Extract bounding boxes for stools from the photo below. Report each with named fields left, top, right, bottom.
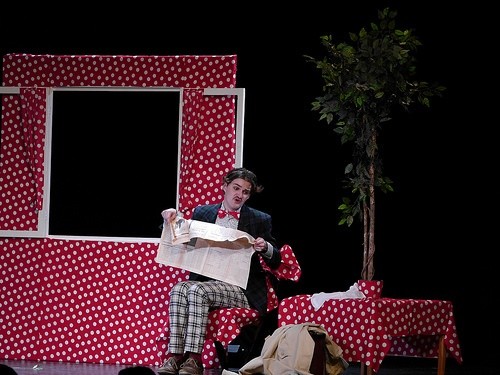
left=308, top=331, right=327, bottom=375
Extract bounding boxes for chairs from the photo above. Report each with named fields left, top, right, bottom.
left=153, top=246, right=300, bottom=375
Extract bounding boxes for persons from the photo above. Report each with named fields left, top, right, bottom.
left=158, top=168, right=282, bottom=375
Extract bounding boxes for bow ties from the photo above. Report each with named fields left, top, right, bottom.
left=217, top=208, right=240, bottom=220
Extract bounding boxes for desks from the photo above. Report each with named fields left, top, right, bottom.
left=278, top=293, right=463, bottom=375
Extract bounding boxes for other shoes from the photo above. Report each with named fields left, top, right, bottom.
left=178, top=357, right=204, bottom=375
left=157, top=355, right=183, bottom=374
